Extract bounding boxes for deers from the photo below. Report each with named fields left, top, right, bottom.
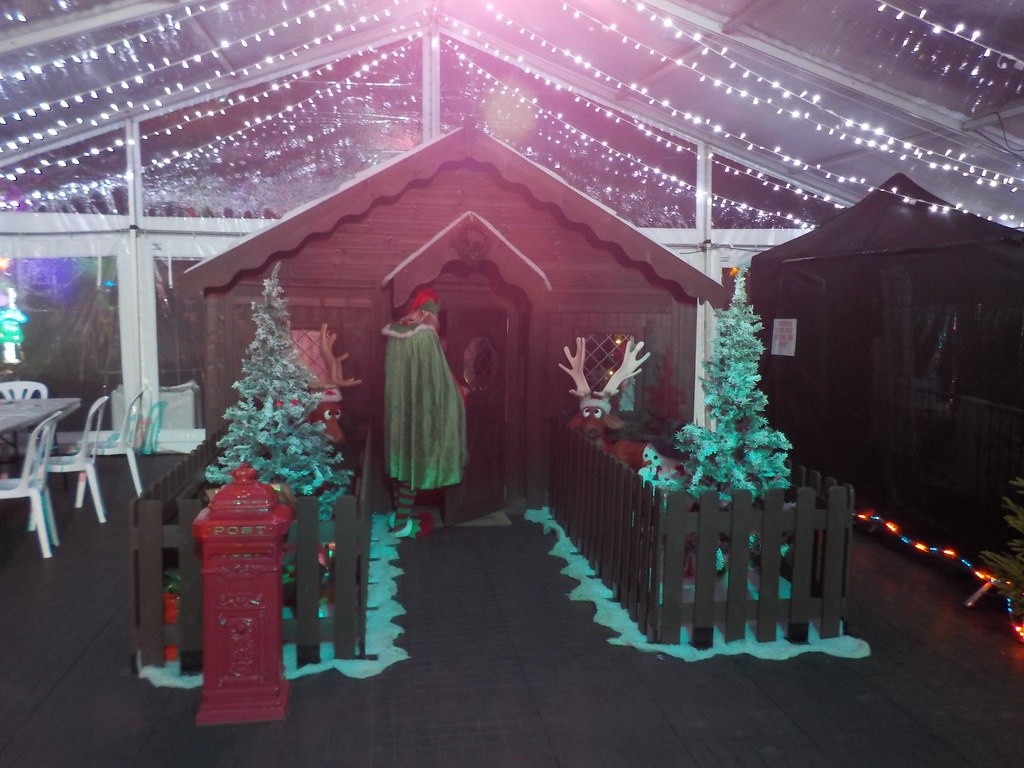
left=277, top=323, right=363, bottom=445
left=557, top=336, right=686, bottom=479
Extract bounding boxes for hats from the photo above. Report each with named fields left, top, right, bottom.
left=412, top=287, right=440, bottom=313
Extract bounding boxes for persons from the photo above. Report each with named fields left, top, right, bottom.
left=381, top=288, right=469, bottom=538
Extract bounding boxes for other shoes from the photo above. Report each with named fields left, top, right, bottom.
left=392, top=513, right=433, bottom=542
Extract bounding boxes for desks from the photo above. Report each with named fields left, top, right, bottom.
left=0, top=398, right=82, bottom=492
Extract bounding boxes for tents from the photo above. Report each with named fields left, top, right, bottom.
left=751, top=173, right=1024, bottom=590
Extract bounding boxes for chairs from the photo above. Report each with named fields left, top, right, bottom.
left=27, top=395, right=110, bottom=532
left=0, top=409, right=64, bottom=559
left=0, top=380, right=49, bottom=454
left=74, top=387, right=147, bottom=509
